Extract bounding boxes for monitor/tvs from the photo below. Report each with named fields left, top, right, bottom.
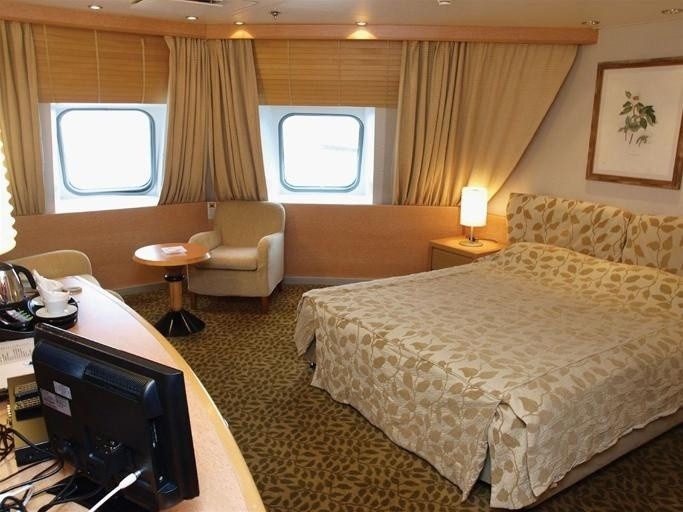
left=31, top=322, right=201, bottom=512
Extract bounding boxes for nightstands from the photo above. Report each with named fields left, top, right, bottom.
left=427, top=234, right=504, bottom=275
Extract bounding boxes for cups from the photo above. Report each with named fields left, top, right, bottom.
left=42, top=291, right=71, bottom=313
left=36, top=286, right=61, bottom=302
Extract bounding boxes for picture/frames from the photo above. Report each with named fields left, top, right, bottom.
left=588, top=56, right=683, bottom=192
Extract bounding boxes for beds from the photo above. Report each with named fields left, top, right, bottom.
left=293, top=193, right=683, bottom=507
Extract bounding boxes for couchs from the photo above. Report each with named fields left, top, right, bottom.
left=184, top=195, right=286, bottom=316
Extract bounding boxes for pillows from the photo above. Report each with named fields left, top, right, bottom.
left=623, top=214, right=683, bottom=277
left=507, top=188, right=636, bottom=267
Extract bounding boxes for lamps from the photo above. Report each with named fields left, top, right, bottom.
left=461, top=186, right=485, bottom=247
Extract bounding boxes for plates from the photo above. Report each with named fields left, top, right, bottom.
left=36, top=305, right=77, bottom=318
left=26, top=295, right=79, bottom=330
left=31, top=296, right=46, bottom=307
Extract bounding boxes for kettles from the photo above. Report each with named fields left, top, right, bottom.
left=0, top=260, right=38, bottom=313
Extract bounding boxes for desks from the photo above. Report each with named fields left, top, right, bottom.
left=0, top=245, right=268, bottom=508
left=131, top=241, right=212, bottom=337
